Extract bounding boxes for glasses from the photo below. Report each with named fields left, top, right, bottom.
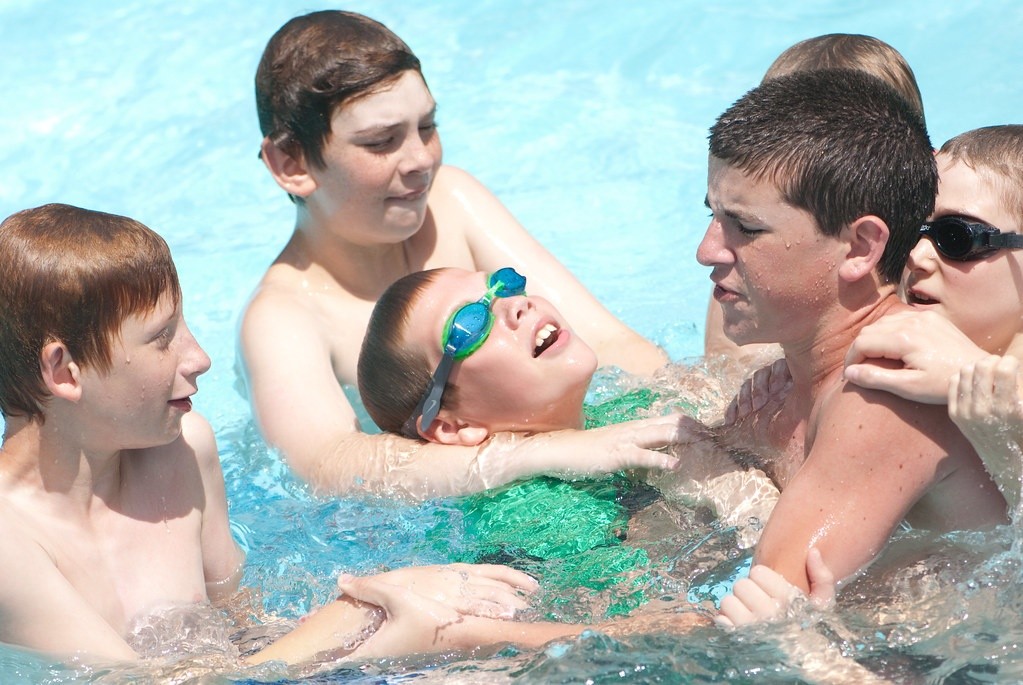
left=918, top=215, right=1023, bottom=262
left=442, top=267, right=527, bottom=361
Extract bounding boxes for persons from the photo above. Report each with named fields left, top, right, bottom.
left=0, top=203, right=540, bottom=669
left=706, top=34, right=1022, bottom=630
left=335, top=69, right=1012, bottom=659
left=358, top=267, right=595, bottom=444
left=241, top=9, right=708, bottom=495
